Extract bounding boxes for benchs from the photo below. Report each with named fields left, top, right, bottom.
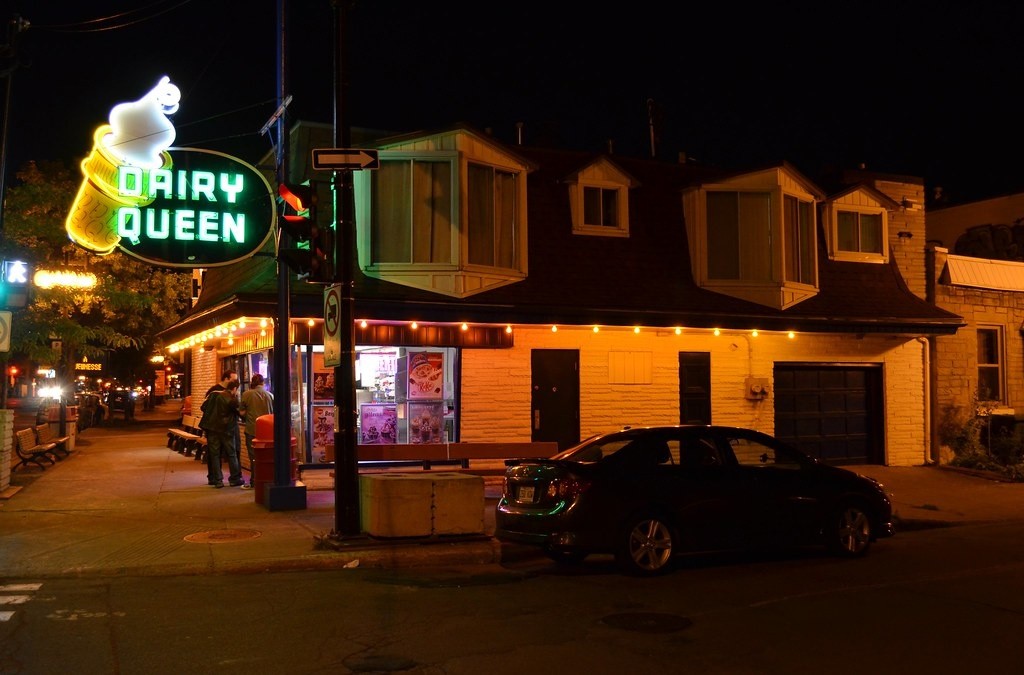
left=33, top=423, right=71, bottom=461
left=325, top=442, right=558, bottom=504
left=11, top=428, right=56, bottom=473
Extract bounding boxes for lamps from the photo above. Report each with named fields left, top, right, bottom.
left=192, top=278, right=201, bottom=299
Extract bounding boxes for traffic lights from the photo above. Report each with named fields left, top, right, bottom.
left=10, top=358, right=17, bottom=375
left=166, top=367, right=172, bottom=377
left=275, top=181, right=338, bottom=284
left=0, top=255, right=33, bottom=312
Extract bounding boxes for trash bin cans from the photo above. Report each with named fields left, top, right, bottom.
left=250, top=414, right=299, bottom=506
left=48, top=405, right=76, bottom=451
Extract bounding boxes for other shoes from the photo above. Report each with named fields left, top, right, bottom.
left=240, top=484, right=255, bottom=489
left=230, top=478, right=245, bottom=486
left=215, top=480, right=224, bottom=487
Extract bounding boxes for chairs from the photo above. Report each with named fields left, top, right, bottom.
left=167, top=415, right=207, bottom=464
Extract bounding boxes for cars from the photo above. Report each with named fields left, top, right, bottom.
left=493, top=425, right=894, bottom=577
left=36, top=390, right=105, bottom=433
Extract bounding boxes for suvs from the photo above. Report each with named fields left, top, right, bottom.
left=108, top=391, right=133, bottom=410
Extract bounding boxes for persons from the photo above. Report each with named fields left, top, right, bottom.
left=203, top=370, right=243, bottom=485
left=239, top=373, right=274, bottom=489
left=198, top=379, right=249, bottom=489
